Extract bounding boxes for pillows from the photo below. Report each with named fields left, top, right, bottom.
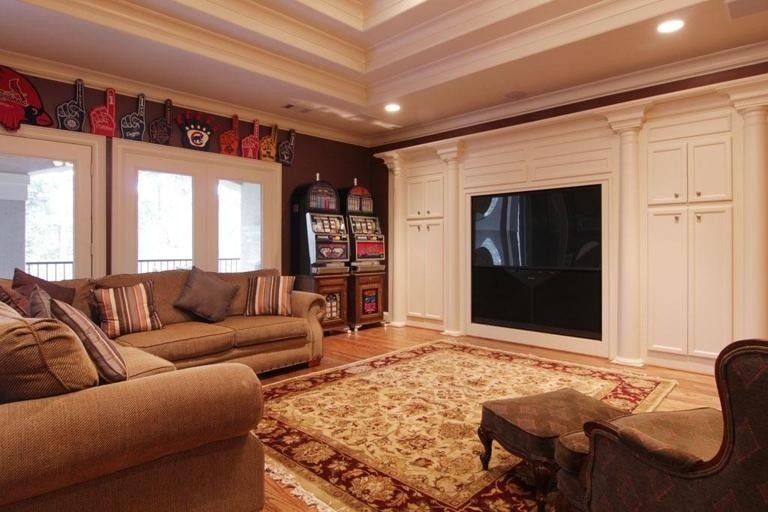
left=0, top=282, right=30, bottom=317
left=173, top=266, right=240, bottom=323
left=27, top=283, right=55, bottom=320
left=243, top=274, right=295, bottom=316
left=91, top=279, right=162, bottom=340
left=47, top=298, right=129, bottom=382
left=12, top=267, right=76, bottom=305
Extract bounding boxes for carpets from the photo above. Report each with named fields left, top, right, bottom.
left=247, top=340, right=678, bottom=511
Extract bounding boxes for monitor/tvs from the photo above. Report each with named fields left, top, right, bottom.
left=472, top=185, right=601, bottom=271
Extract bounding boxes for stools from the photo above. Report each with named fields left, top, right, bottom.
left=477, top=389, right=637, bottom=511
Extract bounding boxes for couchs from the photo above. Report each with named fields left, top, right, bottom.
left=90, top=269, right=327, bottom=375
left=0, top=278, right=264, bottom=512
left=545, top=339, right=768, bottom=512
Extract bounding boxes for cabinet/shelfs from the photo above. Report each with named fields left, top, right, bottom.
left=349, top=272, right=387, bottom=333
left=644, top=132, right=736, bottom=369
left=293, top=275, right=352, bottom=336
left=405, top=173, right=447, bottom=333
